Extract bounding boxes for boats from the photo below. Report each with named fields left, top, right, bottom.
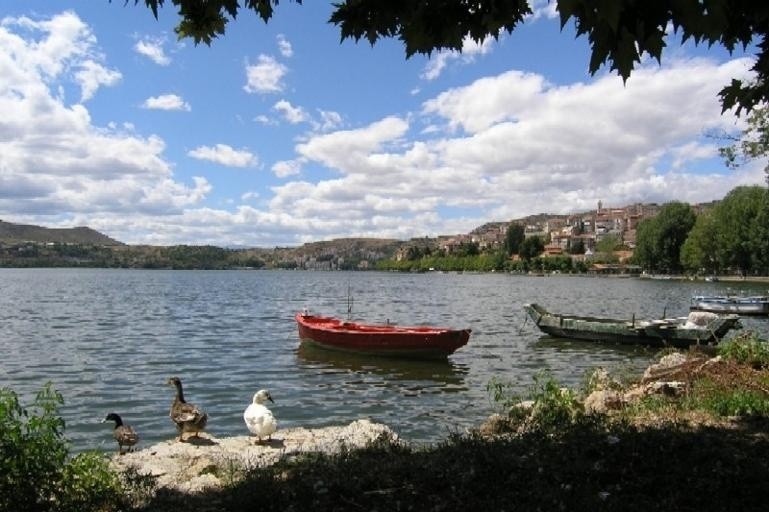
left=293, top=308, right=471, bottom=359
left=520, top=302, right=739, bottom=349
left=690, top=287, right=769, bottom=320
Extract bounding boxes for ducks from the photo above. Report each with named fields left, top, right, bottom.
left=242, top=389, right=279, bottom=445
left=167, top=377, right=207, bottom=443
left=101, top=412, right=140, bottom=454
